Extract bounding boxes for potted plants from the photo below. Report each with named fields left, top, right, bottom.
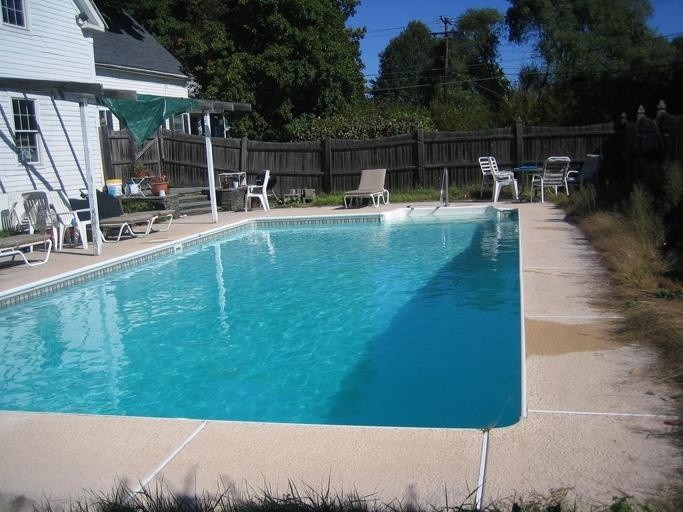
left=134, top=164, right=145, bottom=177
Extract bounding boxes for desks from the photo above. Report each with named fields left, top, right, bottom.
left=512, top=166, right=542, bottom=198
left=219, top=172, right=246, bottom=188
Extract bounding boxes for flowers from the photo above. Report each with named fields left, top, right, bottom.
left=151, top=175, right=168, bottom=183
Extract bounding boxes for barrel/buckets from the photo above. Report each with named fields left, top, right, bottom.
left=106, top=179, right=123, bottom=196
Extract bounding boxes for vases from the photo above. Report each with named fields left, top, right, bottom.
left=151, top=182, right=168, bottom=196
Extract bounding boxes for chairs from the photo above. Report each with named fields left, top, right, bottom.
left=344, top=169, right=389, bottom=207
left=530, top=156, right=570, bottom=204
left=245, top=170, right=270, bottom=212
left=567, top=154, right=601, bottom=191
left=0, top=188, right=175, bottom=266
left=479, top=157, right=518, bottom=203
